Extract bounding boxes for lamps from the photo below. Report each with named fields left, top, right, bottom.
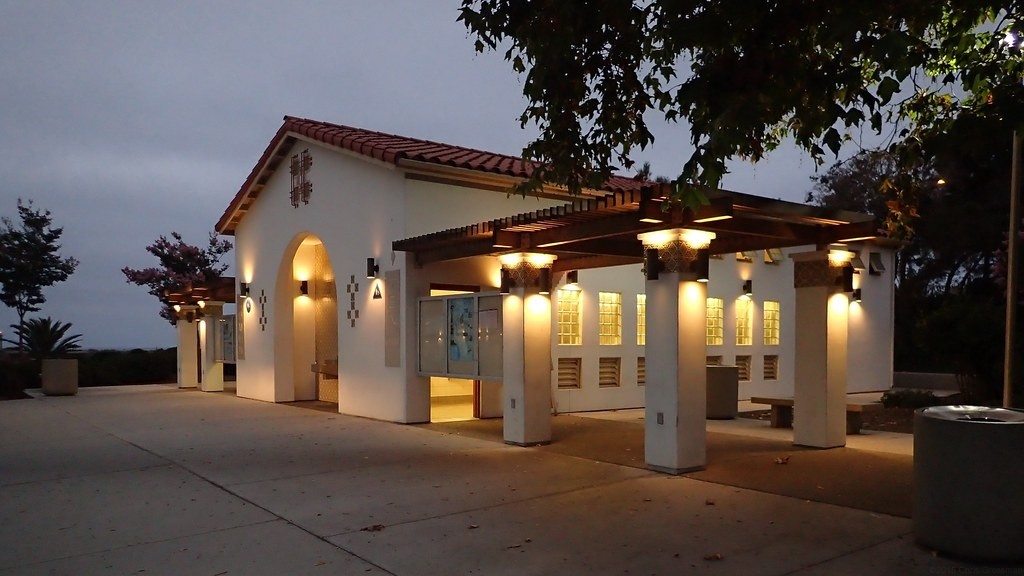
left=366, top=257, right=379, bottom=279
left=568, top=269, right=579, bottom=285
left=538, top=268, right=550, bottom=295
left=647, top=248, right=665, bottom=281
left=195, top=308, right=205, bottom=322
left=841, top=264, right=862, bottom=306
left=299, top=281, right=308, bottom=294
left=499, top=268, right=516, bottom=295
left=240, top=283, right=250, bottom=297
left=742, top=280, right=753, bottom=296
left=691, top=248, right=709, bottom=282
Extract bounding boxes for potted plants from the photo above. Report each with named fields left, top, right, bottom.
left=0, top=315, right=82, bottom=395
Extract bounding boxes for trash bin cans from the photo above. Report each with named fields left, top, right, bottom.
left=706, top=364, right=738, bottom=420
left=911, top=403, right=1024, bottom=563
left=41, top=358, right=78, bottom=394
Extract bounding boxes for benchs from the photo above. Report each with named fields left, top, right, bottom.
left=751, top=397, right=884, bottom=437
